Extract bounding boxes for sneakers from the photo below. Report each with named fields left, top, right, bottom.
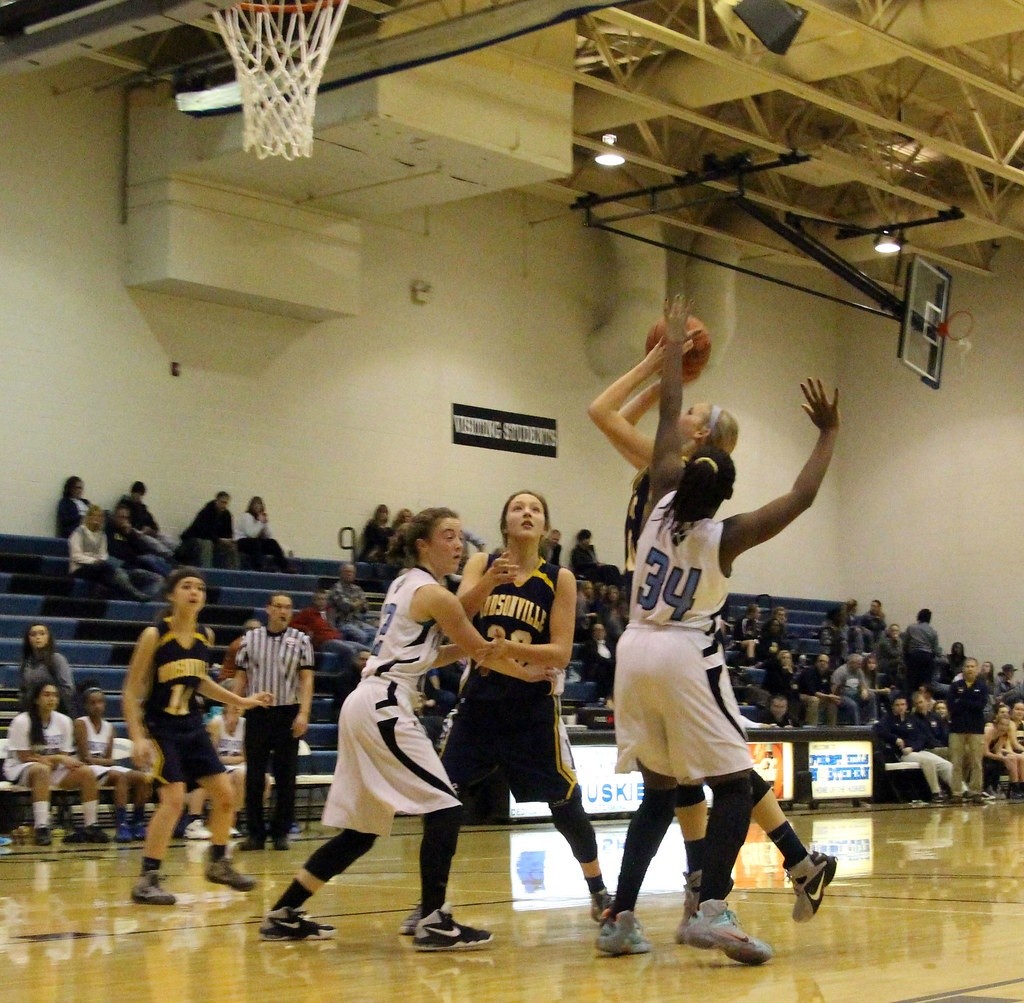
left=595, top=910, right=652, bottom=953
left=274, top=838, right=289, bottom=850
left=132, top=869, right=177, bottom=905
left=784, top=851, right=838, bottom=922
left=206, top=856, right=254, bottom=890
left=677, top=870, right=735, bottom=940
left=684, top=899, right=773, bottom=964
left=35, top=827, right=51, bottom=846
left=239, top=838, right=264, bottom=851
left=411, top=903, right=493, bottom=949
left=260, top=905, right=339, bottom=941
left=589, top=898, right=642, bottom=929
left=117, top=822, right=132, bottom=842
left=82, top=824, right=109, bottom=843
left=133, top=823, right=146, bottom=839
left=399, top=903, right=452, bottom=933
left=184, top=819, right=212, bottom=839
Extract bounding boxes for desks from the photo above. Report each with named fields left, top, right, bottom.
left=483, top=729, right=878, bottom=823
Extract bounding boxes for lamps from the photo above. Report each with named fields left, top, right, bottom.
left=731, top=0, right=811, bottom=59
left=171, top=60, right=243, bottom=117
left=874, top=229, right=900, bottom=255
left=593, top=131, right=626, bottom=167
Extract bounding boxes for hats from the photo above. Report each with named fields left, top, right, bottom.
left=1003, top=664, right=1017, bottom=672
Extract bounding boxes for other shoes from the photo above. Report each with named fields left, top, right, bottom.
left=290, top=824, right=301, bottom=834
left=0, top=837, right=12, bottom=846
left=931, top=785, right=1023, bottom=804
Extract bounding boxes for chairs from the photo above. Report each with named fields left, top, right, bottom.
left=294, top=740, right=335, bottom=832
left=869, top=720, right=922, bottom=803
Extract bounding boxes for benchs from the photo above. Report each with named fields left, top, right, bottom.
left=0, top=532, right=863, bottom=770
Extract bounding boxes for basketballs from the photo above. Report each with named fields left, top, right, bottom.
left=644, top=313, right=711, bottom=383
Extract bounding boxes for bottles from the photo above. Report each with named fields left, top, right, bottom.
left=289, top=550, right=293, bottom=558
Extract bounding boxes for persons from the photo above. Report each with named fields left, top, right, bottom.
left=259, top=509, right=559, bottom=949
left=226, top=595, right=313, bottom=851
left=415, top=529, right=487, bottom=747
left=4, top=617, right=309, bottom=844
left=721, top=595, right=1024, bottom=805
left=589, top=327, right=836, bottom=941
left=330, top=652, right=370, bottom=721
left=290, top=563, right=377, bottom=666
left=233, top=495, right=299, bottom=573
left=122, top=568, right=274, bottom=904
left=57, top=475, right=219, bottom=602
left=184, top=493, right=241, bottom=570
left=595, top=292, right=839, bottom=963
left=360, top=504, right=415, bottom=590
left=544, top=527, right=631, bottom=704
left=402, top=491, right=642, bottom=934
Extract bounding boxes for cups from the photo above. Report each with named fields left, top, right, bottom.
left=566, top=716, right=577, bottom=725
